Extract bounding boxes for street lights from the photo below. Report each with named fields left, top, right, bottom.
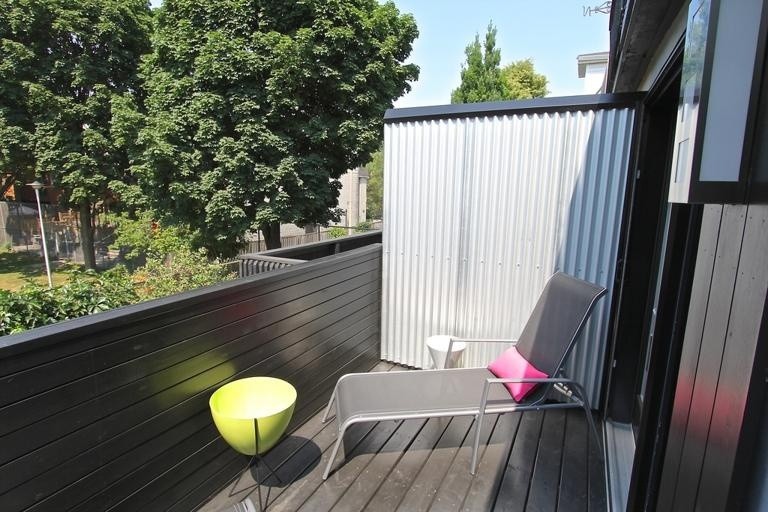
left=23, top=179, right=55, bottom=292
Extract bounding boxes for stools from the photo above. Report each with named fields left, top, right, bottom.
left=208, top=375, right=297, bottom=512
left=426, top=334, right=465, bottom=368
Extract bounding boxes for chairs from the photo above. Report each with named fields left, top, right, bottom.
left=322, top=273, right=606, bottom=479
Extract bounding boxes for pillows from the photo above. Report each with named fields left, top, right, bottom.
left=487, top=343, right=548, bottom=403
